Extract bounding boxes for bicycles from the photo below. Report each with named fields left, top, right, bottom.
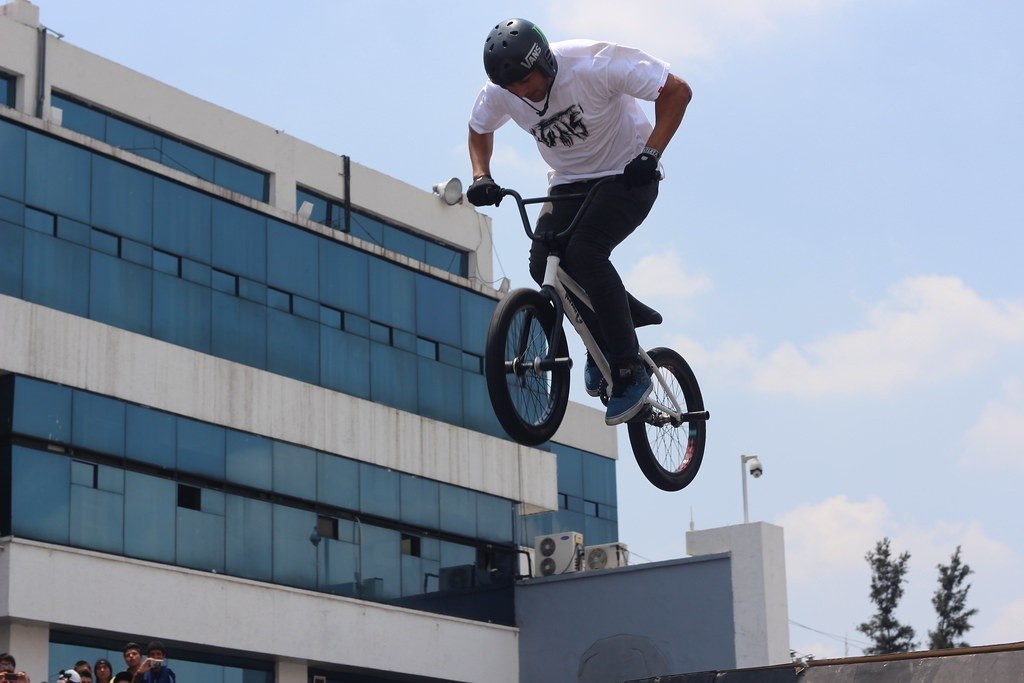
left=485, top=168, right=715, bottom=495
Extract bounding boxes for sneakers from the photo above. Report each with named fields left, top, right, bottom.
left=584, top=346, right=606, bottom=397
left=605, top=365, right=654, bottom=425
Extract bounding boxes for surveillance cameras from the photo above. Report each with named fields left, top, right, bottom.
left=749, top=460, right=764, bottom=478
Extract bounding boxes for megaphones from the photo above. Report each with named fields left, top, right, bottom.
left=433, top=177, right=463, bottom=205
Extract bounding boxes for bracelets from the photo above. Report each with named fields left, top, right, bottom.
left=642, top=147, right=661, bottom=161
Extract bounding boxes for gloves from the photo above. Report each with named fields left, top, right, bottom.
left=467, top=174, right=500, bottom=207
left=624, top=147, right=662, bottom=184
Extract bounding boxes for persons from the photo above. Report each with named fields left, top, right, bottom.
left=59, top=642, right=175, bottom=683
left=0, top=653, right=29, bottom=683
left=468, top=18, right=693, bottom=426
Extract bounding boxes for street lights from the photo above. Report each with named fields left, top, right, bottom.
left=741, top=453, right=764, bottom=523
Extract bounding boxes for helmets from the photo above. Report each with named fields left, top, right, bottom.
left=484, top=18, right=557, bottom=85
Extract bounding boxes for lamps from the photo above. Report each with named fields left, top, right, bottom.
left=50, top=104, right=64, bottom=127
left=498, top=277, right=511, bottom=294
left=298, top=200, right=315, bottom=219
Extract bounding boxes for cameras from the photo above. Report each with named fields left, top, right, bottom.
left=150, top=659, right=165, bottom=666
left=4, top=673, right=19, bottom=680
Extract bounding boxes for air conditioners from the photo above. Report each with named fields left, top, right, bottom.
left=439, top=565, right=475, bottom=589
left=535, top=530, right=585, bottom=578
left=582, top=542, right=628, bottom=570
